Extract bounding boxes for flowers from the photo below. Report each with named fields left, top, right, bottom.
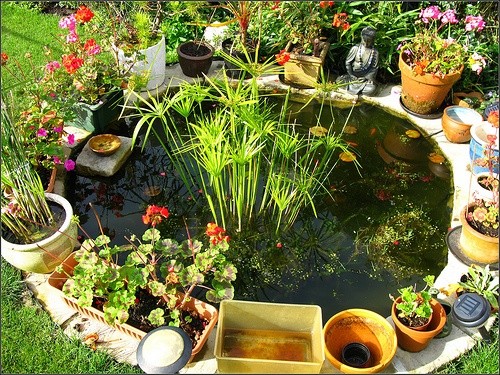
left=0, top=1, right=150, bottom=236
left=186, top=1, right=349, bottom=55
left=464, top=111, right=499, bottom=230
left=1, top=201, right=238, bottom=327
left=398, top=3, right=489, bottom=78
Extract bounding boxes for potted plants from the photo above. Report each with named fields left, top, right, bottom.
left=440, top=262, right=499, bottom=312
left=169, top=1, right=216, bottom=78
left=389, top=273, right=440, bottom=330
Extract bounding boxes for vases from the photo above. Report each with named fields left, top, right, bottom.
left=47, top=251, right=218, bottom=363
left=47, top=79, right=125, bottom=134
left=442, top=104, right=485, bottom=144
left=471, top=170, right=500, bottom=203
left=322, top=307, right=398, bottom=375
left=3, top=113, right=65, bottom=198
left=391, top=294, right=447, bottom=354
left=222, top=36, right=260, bottom=78
left=468, top=120, right=499, bottom=175
left=110, top=28, right=166, bottom=92
left=1, top=194, right=77, bottom=274
left=460, top=199, right=499, bottom=264
left=283, top=38, right=330, bottom=87
left=398, top=49, right=466, bottom=113
